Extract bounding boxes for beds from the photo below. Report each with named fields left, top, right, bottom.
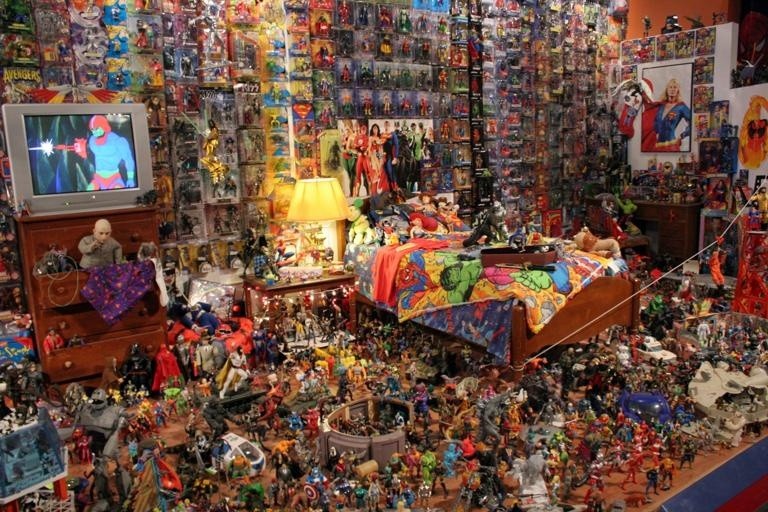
left=338, top=191, right=641, bottom=384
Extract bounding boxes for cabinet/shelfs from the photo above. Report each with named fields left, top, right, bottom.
left=13, top=203, right=169, bottom=392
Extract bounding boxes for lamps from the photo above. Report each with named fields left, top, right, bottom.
left=286, top=177, right=352, bottom=272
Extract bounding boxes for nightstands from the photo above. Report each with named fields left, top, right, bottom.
left=242, top=273, right=356, bottom=336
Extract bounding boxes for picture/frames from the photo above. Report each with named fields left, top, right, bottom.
left=641, top=62, right=694, bottom=153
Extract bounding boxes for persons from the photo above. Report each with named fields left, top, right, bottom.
left=1, top=0, right=768, bottom=512
left=0, top=1, right=767, bottom=511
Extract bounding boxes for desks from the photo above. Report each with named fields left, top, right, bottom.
left=621, top=198, right=703, bottom=263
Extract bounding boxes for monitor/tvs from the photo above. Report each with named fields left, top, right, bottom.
left=1, top=103, right=154, bottom=217
